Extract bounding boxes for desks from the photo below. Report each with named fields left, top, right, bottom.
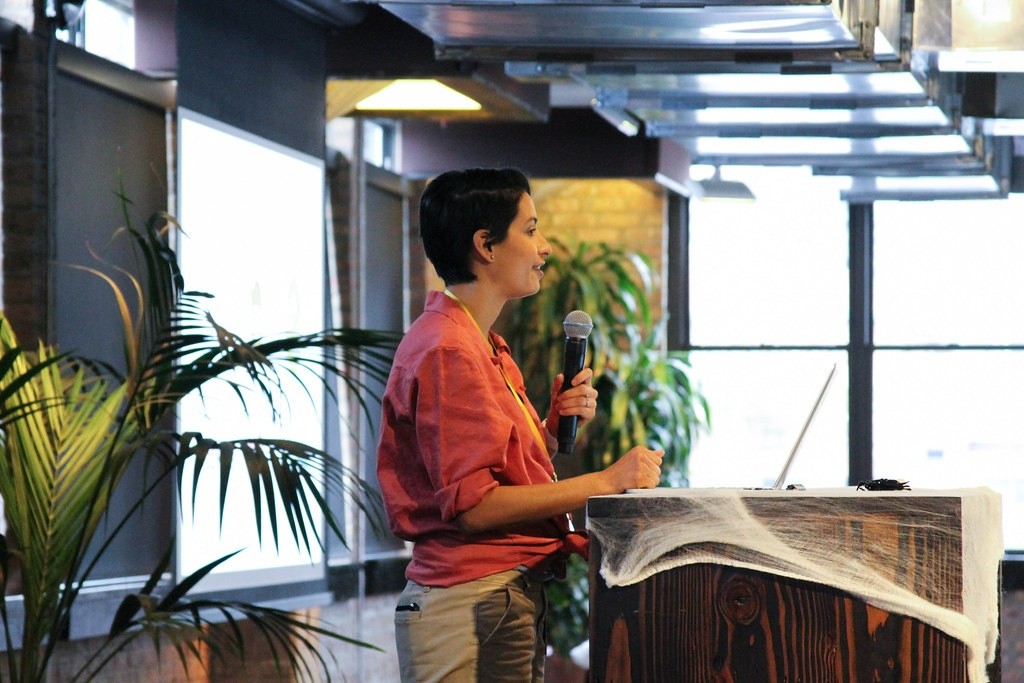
left=586, top=485, right=1006, bottom=683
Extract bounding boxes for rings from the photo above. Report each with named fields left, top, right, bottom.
left=584, top=394, right=589, bottom=408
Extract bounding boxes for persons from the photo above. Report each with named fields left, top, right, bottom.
left=374, top=168, right=667, bottom=683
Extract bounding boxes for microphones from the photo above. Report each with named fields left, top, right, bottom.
left=557, top=310, right=593, bottom=456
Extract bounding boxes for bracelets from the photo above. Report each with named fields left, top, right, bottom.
left=541, top=418, right=558, bottom=452
